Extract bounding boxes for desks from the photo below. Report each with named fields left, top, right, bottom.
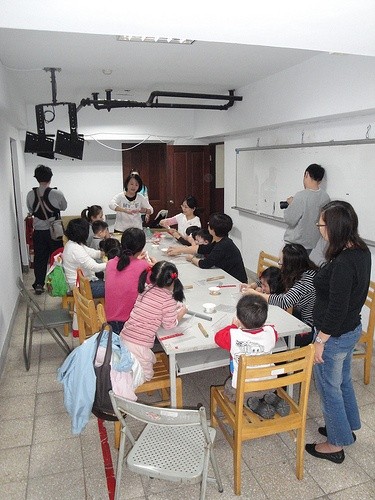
left=113, top=231, right=312, bottom=409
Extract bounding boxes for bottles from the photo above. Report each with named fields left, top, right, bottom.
left=146, top=227, right=150, bottom=237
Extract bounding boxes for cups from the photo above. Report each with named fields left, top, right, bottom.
left=203, top=303, right=216, bottom=314
left=162, top=248, right=168, bottom=255
left=209, top=287, right=221, bottom=295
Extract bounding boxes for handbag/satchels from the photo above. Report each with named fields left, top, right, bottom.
left=45, top=266, right=68, bottom=297
left=87, top=324, right=128, bottom=422
left=49, top=219, right=66, bottom=241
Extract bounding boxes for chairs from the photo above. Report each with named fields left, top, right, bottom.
left=256, top=251, right=282, bottom=276
left=17, top=276, right=73, bottom=370
left=108, top=390, right=224, bottom=500
left=211, top=343, right=316, bottom=495
left=353, top=280, right=375, bottom=384
left=97, top=303, right=183, bottom=448
left=57, top=257, right=102, bottom=372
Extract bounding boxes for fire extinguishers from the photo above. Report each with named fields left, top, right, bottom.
left=23, top=212, right=35, bottom=252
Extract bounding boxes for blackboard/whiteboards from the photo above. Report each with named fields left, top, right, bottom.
left=229, top=137, right=375, bottom=248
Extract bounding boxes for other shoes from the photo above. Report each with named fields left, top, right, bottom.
left=319, top=425, right=357, bottom=444
left=247, top=396, right=276, bottom=419
left=305, top=444, right=345, bottom=463
left=263, top=393, right=291, bottom=417
left=34, top=284, right=45, bottom=294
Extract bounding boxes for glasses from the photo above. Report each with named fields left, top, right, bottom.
left=181, top=205, right=192, bottom=209
left=278, top=259, right=284, bottom=265
left=315, top=222, right=327, bottom=229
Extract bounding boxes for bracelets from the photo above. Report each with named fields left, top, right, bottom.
left=316, top=337, right=321, bottom=343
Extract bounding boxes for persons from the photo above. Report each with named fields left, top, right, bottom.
left=195, top=229, right=213, bottom=259
left=303, top=201, right=372, bottom=462
left=27, top=166, right=67, bottom=295
left=81, top=205, right=103, bottom=247
left=215, top=294, right=290, bottom=419
left=240, top=244, right=316, bottom=348
left=110, top=174, right=153, bottom=233
left=119, top=261, right=186, bottom=381
left=124, top=169, right=150, bottom=228
left=260, top=266, right=293, bottom=315
left=106, top=228, right=153, bottom=336
left=283, top=164, right=329, bottom=255
left=63, top=218, right=105, bottom=298
left=167, top=213, right=248, bottom=284
left=161, top=196, right=201, bottom=246
left=174, top=226, right=200, bottom=246
left=90, top=220, right=108, bottom=263
